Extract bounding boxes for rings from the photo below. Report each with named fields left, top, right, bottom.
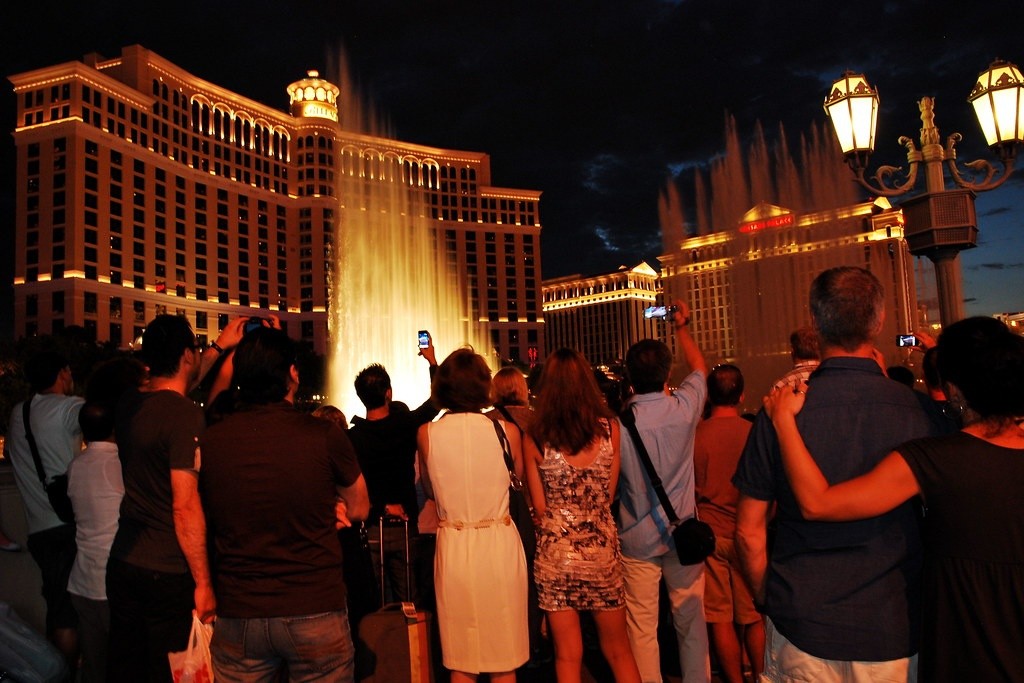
left=796, top=391, right=806, bottom=395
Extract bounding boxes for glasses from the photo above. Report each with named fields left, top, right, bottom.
left=192, top=344, right=203, bottom=354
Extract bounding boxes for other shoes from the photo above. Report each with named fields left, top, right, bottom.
left=0, top=539, right=21, bottom=552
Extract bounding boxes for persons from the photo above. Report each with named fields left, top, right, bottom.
left=417, top=348, right=538, bottom=683
left=610, top=300, right=711, bottom=683
left=198, top=326, right=369, bottom=683
left=770, top=324, right=956, bottom=416
left=521, top=348, right=642, bottom=683
left=8, top=315, right=348, bottom=682
left=692, top=363, right=767, bottom=682
left=105, top=314, right=249, bottom=683
left=763, top=316, right=1024, bottom=683
left=730, top=267, right=959, bottom=683
left=348, top=331, right=440, bottom=614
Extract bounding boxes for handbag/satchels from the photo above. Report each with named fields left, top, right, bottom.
left=168, top=609, right=215, bottom=682
left=48, top=477, right=74, bottom=523
left=672, top=519, right=715, bottom=566
left=510, top=484, right=536, bottom=565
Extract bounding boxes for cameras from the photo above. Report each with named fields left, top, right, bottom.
left=642, top=304, right=680, bottom=321
left=243, top=317, right=273, bottom=335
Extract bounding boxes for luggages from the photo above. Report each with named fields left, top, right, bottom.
left=357, top=515, right=448, bottom=682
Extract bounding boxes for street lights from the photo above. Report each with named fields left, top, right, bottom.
left=823, top=56, right=1024, bottom=331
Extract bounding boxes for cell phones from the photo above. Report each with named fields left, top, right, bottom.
left=896, top=335, right=919, bottom=347
left=418, top=330, right=429, bottom=349
